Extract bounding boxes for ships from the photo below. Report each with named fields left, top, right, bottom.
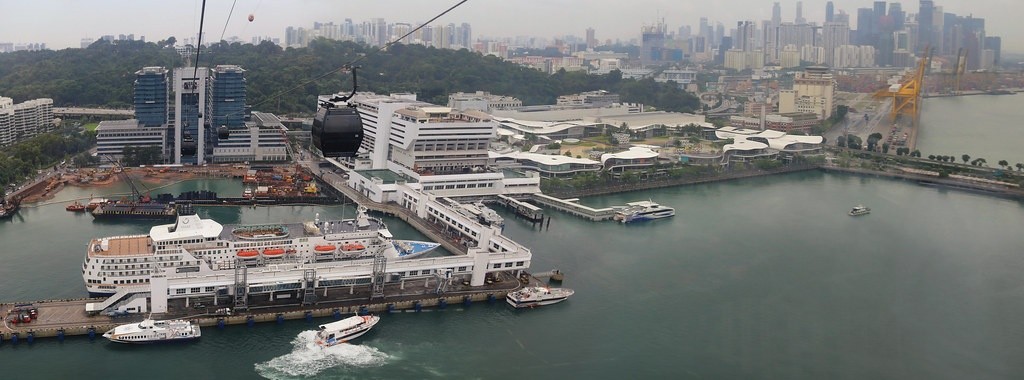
left=79, top=212, right=441, bottom=298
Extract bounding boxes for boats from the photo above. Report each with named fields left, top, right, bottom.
left=847, top=204, right=872, bottom=218
left=66, top=202, right=86, bottom=212
left=101, top=319, right=202, bottom=345
left=221, top=216, right=389, bottom=240
left=505, top=286, right=575, bottom=309
left=613, top=199, right=677, bottom=224
left=312, top=314, right=381, bottom=350
